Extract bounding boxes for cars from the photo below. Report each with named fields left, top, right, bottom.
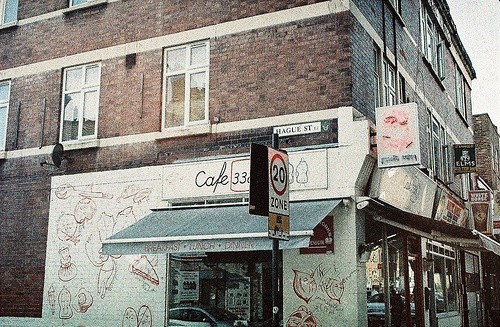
left=166, top=304, right=249, bottom=327
